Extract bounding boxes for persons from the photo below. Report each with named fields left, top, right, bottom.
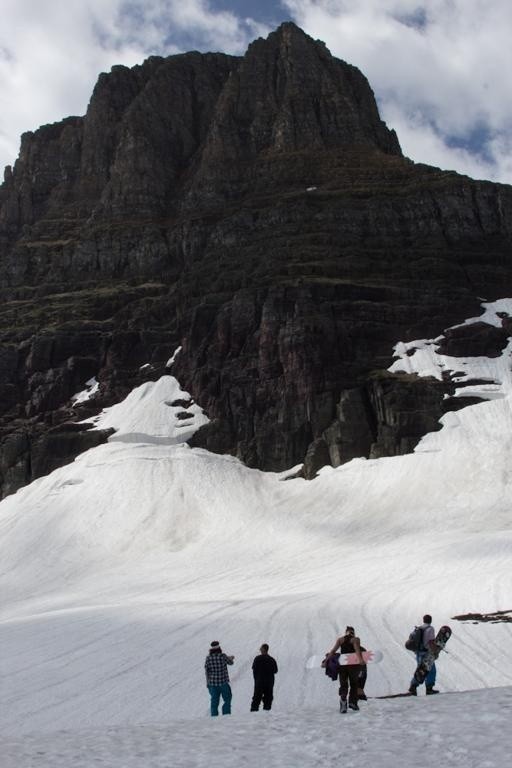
left=324, top=626, right=364, bottom=713
left=250, top=643, right=278, bottom=711
left=408, top=614, right=439, bottom=695
left=204, top=640, right=235, bottom=715
left=353, top=638, right=367, bottom=701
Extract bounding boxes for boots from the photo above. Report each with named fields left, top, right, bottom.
left=340, top=699, right=347, bottom=713
left=349, top=702, right=359, bottom=710
left=426, top=686, right=439, bottom=694
left=409, top=686, right=417, bottom=695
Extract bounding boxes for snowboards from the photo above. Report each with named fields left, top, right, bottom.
left=307, top=651, right=382, bottom=668
left=414, top=626, right=452, bottom=684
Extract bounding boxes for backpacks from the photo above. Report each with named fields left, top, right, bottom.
left=405, top=626, right=430, bottom=650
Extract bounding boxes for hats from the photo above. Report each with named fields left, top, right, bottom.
left=210, top=642, right=219, bottom=648
